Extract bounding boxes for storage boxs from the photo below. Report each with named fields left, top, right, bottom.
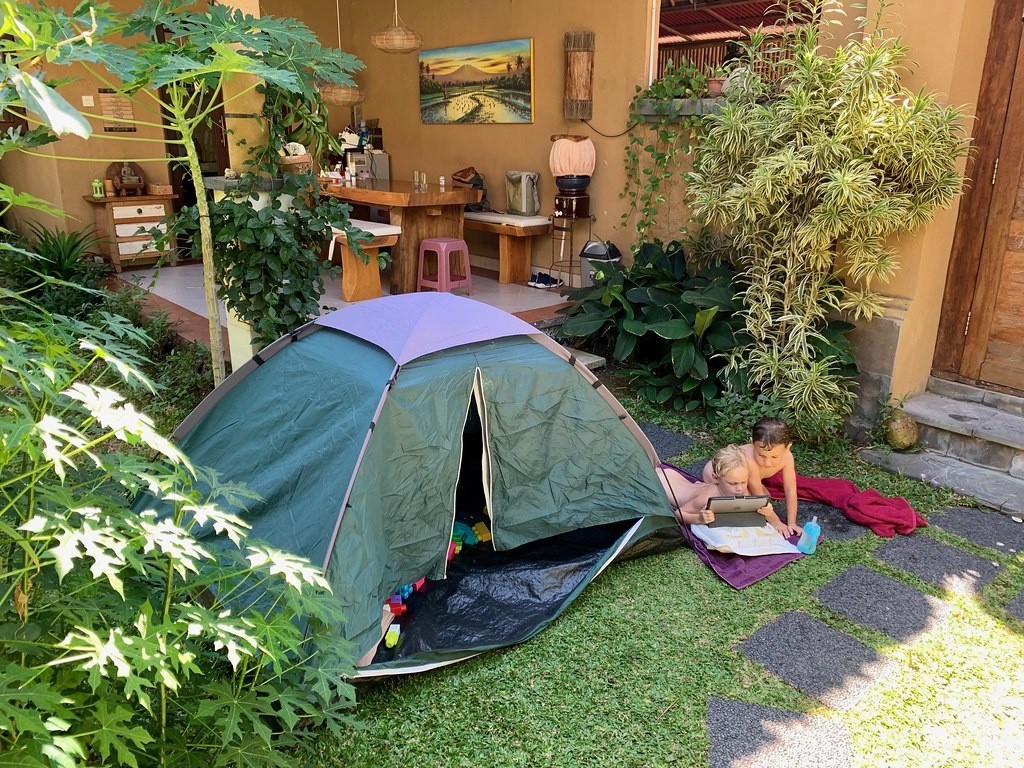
left=342, top=130, right=360, bottom=145
left=276, top=154, right=311, bottom=175
left=368, top=128, right=383, bottom=150
left=146, top=182, right=174, bottom=195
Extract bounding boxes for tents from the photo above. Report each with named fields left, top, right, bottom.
left=130, top=291, right=685, bottom=679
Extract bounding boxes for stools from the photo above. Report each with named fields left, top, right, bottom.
left=416, top=236, right=474, bottom=297
left=549, top=215, right=592, bottom=294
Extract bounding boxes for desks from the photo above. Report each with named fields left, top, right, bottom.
left=310, top=177, right=483, bottom=297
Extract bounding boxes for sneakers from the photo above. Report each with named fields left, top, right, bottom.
left=528, top=273, right=538, bottom=287
left=535, top=272, right=563, bottom=288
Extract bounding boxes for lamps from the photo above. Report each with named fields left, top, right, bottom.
left=561, top=29, right=595, bottom=121
left=91, top=179, right=106, bottom=199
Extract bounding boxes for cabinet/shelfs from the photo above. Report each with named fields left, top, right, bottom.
left=82, top=193, right=181, bottom=274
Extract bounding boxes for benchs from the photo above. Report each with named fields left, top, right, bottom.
left=321, top=217, right=402, bottom=303
left=462, top=211, right=552, bottom=285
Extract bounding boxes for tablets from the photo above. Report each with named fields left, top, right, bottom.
left=706, top=495, right=770, bottom=515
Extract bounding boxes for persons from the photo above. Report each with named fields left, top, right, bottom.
left=655, top=444, right=773, bottom=524
left=702, top=416, right=802, bottom=540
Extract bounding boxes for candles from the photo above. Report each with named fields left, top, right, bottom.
left=413, top=170, right=419, bottom=181
left=421, top=172, right=426, bottom=184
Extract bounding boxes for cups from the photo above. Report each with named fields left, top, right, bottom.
left=413, top=171, right=427, bottom=190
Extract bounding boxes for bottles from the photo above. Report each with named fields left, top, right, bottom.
left=796, top=516, right=820, bottom=554
left=344, top=167, right=350, bottom=181
left=440, top=176, right=445, bottom=186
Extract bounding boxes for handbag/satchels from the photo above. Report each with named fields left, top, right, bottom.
left=505, top=170, right=541, bottom=216
left=451, top=166, right=504, bottom=215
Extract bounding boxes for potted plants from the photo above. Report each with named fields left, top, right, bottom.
left=705, top=64, right=731, bottom=98
left=720, top=20, right=784, bottom=97
